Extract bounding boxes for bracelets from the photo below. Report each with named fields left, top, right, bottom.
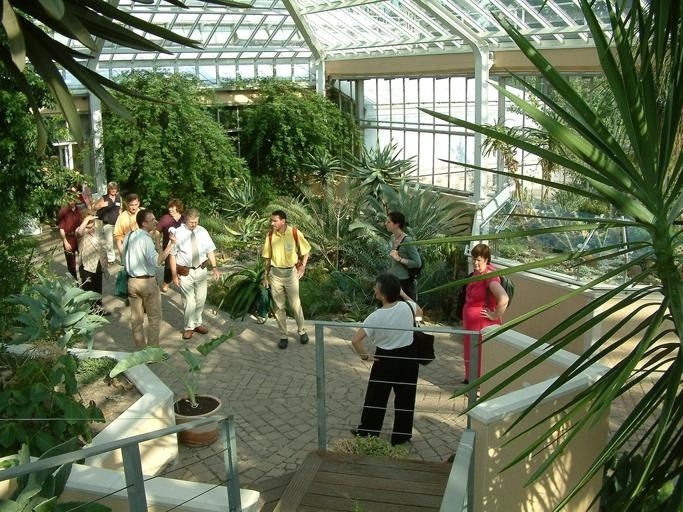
left=299, top=262, right=305, bottom=267
left=211, top=265, right=216, bottom=268
left=361, top=355, right=368, bottom=360
left=398, top=256, right=404, bottom=264
left=262, top=273, right=267, bottom=277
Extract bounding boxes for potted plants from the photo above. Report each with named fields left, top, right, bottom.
left=108, top=328, right=234, bottom=448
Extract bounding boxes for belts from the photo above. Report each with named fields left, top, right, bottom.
left=189, top=264, right=201, bottom=269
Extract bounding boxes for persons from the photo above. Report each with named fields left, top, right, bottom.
left=460, top=244, right=509, bottom=398
left=122, top=209, right=176, bottom=365
left=350, top=275, right=422, bottom=450
left=260, top=210, right=312, bottom=350
left=58, top=180, right=122, bottom=324
left=112, top=191, right=153, bottom=312
left=383, top=212, right=423, bottom=300
left=166, top=208, right=219, bottom=340
left=154, top=198, right=186, bottom=293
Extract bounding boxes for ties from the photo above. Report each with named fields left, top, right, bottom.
left=190, top=230, right=199, bottom=269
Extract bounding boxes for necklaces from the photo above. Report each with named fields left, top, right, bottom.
left=392, top=233, right=403, bottom=249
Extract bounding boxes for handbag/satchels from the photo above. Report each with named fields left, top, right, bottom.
left=115, top=270, right=128, bottom=298
left=96, top=207, right=109, bottom=224
left=404, top=300, right=435, bottom=366
left=396, top=236, right=425, bottom=280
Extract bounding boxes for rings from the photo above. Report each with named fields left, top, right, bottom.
left=485, top=310, right=487, bottom=313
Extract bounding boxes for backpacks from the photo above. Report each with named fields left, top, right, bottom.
left=269, top=224, right=302, bottom=269
left=466, top=272, right=514, bottom=308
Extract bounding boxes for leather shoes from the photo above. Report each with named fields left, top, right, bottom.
left=278, top=339, right=287, bottom=349
left=350, top=429, right=362, bottom=438
left=193, top=326, right=207, bottom=334
left=300, top=332, right=308, bottom=344
left=161, top=281, right=169, bottom=292
left=182, top=330, right=192, bottom=339
